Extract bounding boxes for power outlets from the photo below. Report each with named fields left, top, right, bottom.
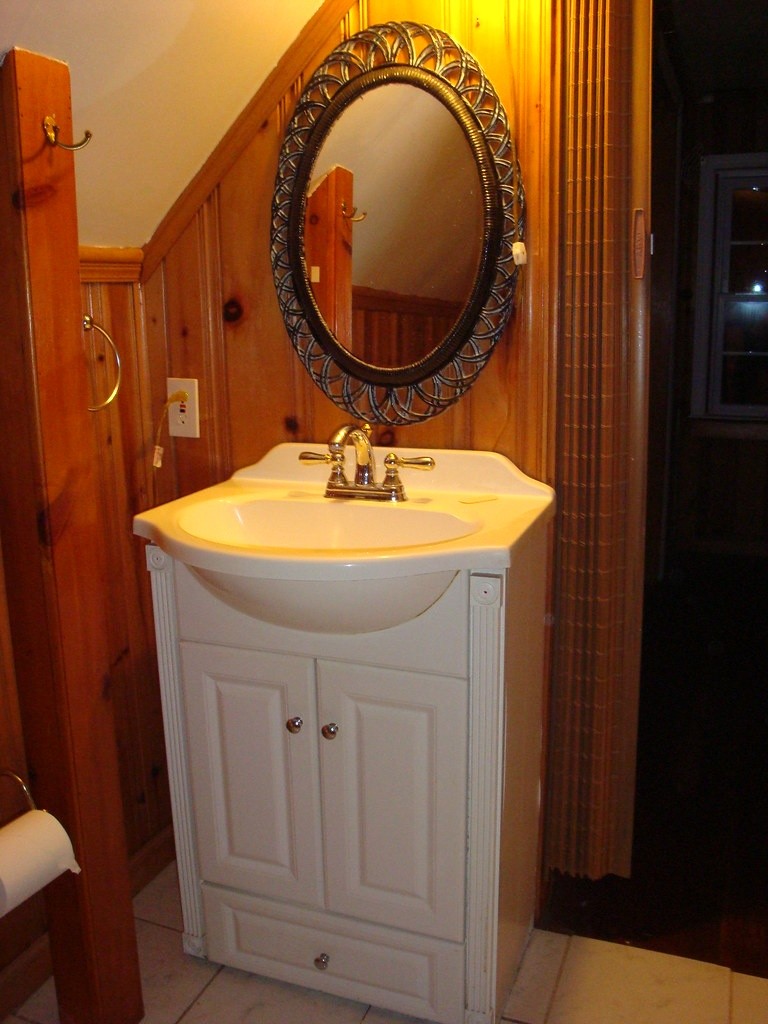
left=165, top=377, right=199, bottom=439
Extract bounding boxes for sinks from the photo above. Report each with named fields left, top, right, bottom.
left=131, top=442, right=561, bottom=636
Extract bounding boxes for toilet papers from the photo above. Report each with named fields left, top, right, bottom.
left=0, top=808, right=83, bottom=920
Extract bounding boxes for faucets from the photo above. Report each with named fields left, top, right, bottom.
left=297, top=422, right=438, bottom=504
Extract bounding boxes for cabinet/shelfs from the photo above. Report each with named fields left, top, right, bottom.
left=140, top=543, right=550, bottom=1024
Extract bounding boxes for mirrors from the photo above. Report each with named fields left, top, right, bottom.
left=268, top=20, right=527, bottom=424
